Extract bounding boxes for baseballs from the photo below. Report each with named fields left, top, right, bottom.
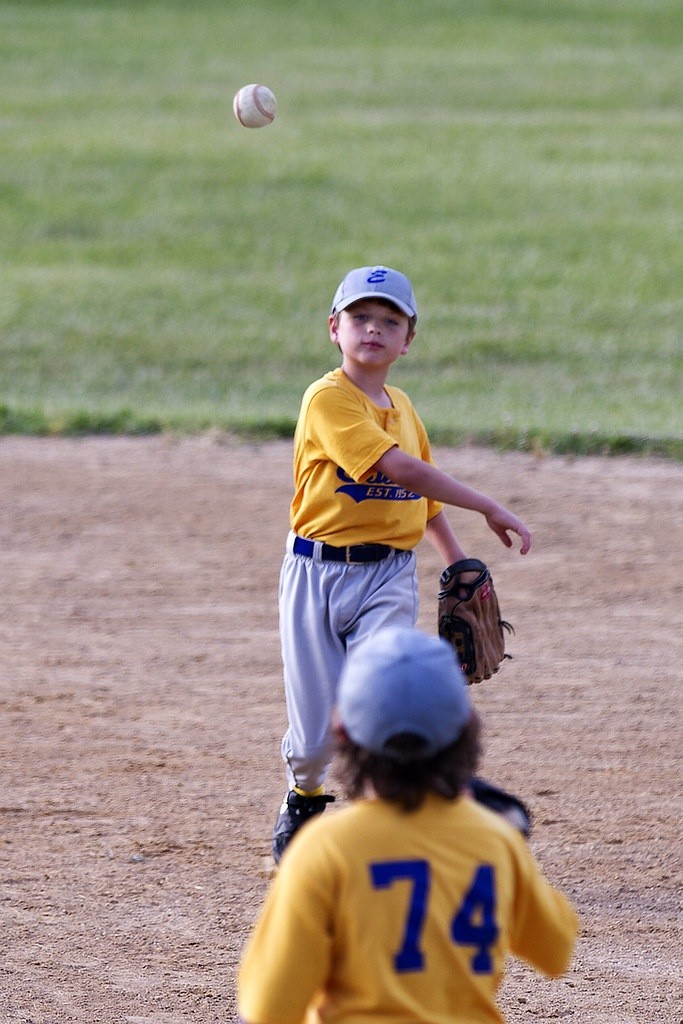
left=234, top=83, right=278, bottom=129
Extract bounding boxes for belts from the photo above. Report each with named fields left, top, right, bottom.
left=294, top=535, right=405, bottom=566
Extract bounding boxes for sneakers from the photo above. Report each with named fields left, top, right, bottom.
left=272, top=790, right=335, bottom=867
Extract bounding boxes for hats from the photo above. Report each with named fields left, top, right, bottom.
left=338, top=625, right=470, bottom=757
left=332, top=266, right=418, bottom=330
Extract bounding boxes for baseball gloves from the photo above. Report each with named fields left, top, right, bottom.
left=439, top=557, right=505, bottom=685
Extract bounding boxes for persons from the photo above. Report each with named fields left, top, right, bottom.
left=236, top=627, right=575, bottom=1024
left=275, top=266, right=532, bottom=870
